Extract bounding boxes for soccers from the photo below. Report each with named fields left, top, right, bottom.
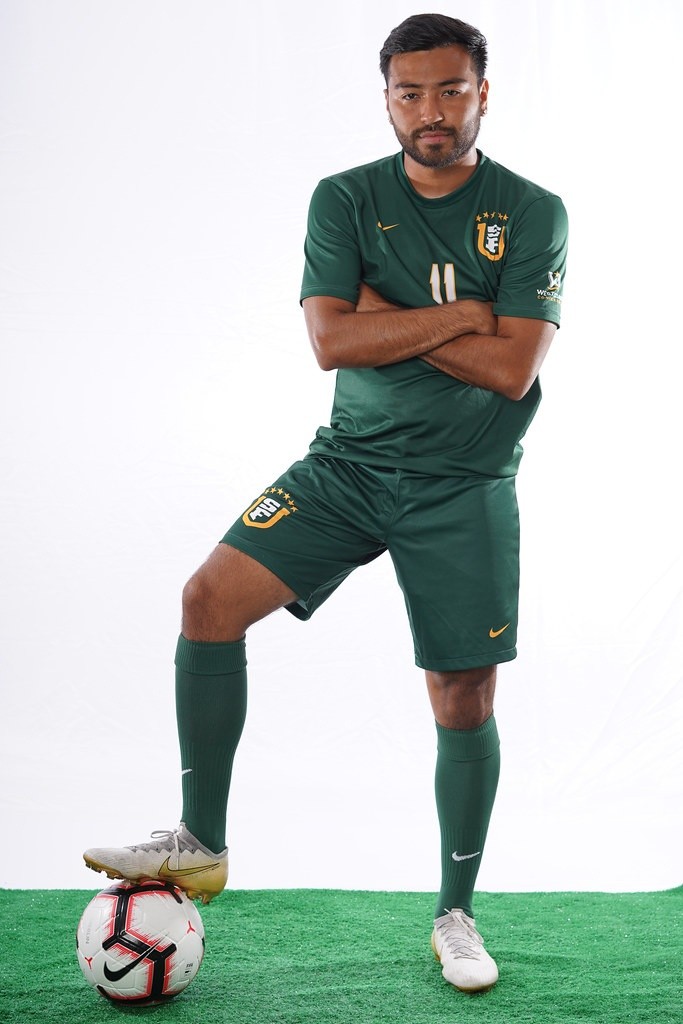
left=75, top=877, right=208, bottom=1006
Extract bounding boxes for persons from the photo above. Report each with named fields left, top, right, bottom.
left=81, top=15, right=569, bottom=992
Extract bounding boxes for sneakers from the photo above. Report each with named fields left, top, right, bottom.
left=82, top=820, right=230, bottom=906
left=430, top=907, right=500, bottom=993
